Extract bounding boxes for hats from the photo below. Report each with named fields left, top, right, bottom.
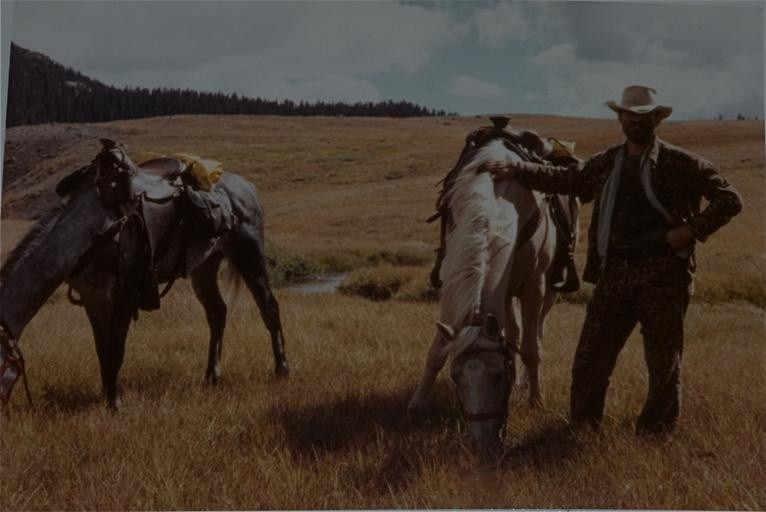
left=605, top=86, right=671, bottom=118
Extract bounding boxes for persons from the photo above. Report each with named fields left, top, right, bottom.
left=472, top=84, right=746, bottom=447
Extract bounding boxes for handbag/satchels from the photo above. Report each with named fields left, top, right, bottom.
left=183, top=186, right=239, bottom=237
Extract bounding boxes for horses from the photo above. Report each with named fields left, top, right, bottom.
left=0, top=166, right=291, bottom=417
left=401, top=137, right=581, bottom=466
